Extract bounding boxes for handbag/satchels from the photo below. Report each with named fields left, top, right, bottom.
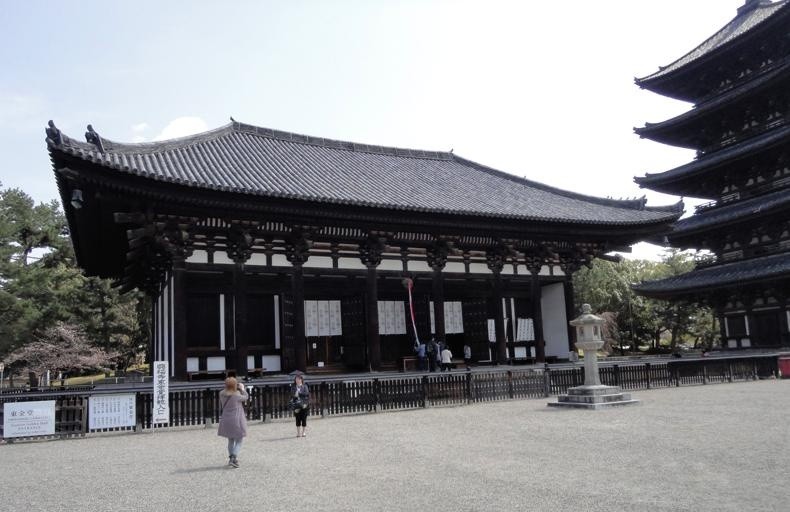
left=290, top=398, right=302, bottom=409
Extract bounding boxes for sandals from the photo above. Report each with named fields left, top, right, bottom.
left=297, top=432, right=307, bottom=437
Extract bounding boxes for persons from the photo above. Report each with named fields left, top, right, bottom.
left=414, top=337, right=452, bottom=372
left=290, top=376, right=310, bottom=437
left=464, top=343, right=472, bottom=371
left=217, top=377, right=249, bottom=468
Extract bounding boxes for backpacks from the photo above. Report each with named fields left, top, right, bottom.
left=427, top=343, right=433, bottom=352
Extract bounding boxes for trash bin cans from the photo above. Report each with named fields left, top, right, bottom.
left=778, top=357, right=790, bottom=377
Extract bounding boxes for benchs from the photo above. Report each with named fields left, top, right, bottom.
left=184, top=368, right=268, bottom=382
left=492, top=356, right=552, bottom=365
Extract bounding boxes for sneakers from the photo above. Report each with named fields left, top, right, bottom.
left=467, top=366, right=472, bottom=371
left=416, top=367, right=453, bottom=372
left=228, top=455, right=241, bottom=468
